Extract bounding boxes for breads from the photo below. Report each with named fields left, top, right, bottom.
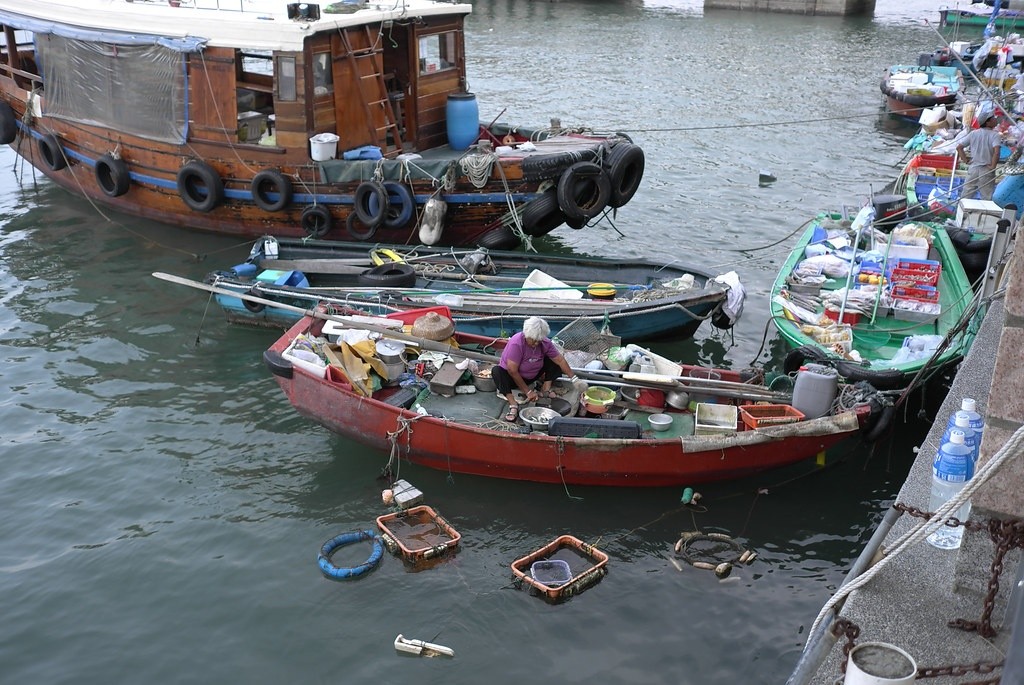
left=801, top=318, right=849, bottom=343
left=859, top=274, right=888, bottom=284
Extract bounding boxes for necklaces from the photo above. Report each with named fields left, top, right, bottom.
left=530, top=347, right=536, bottom=354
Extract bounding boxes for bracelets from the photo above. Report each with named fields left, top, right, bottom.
left=571, top=375, right=579, bottom=383
left=526, top=390, right=532, bottom=395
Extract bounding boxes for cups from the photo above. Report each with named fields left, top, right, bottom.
left=948, top=399, right=984, bottom=449
left=925, top=431, right=977, bottom=549
left=937, top=414, right=980, bottom=493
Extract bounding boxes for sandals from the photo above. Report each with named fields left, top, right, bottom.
left=505, top=401, right=519, bottom=422
left=538, top=389, right=560, bottom=400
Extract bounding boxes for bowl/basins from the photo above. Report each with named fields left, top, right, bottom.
left=470, top=364, right=498, bottom=392
left=580, top=386, right=616, bottom=412
left=666, top=391, right=689, bottom=410
left=648, top=413, right=674, bottom=431
left=518, top=407, right=562, bottom=430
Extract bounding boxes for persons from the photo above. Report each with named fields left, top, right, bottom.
left=491, top=317, right=588, bottom=423
left=956, top=111, right=1002, bottom=200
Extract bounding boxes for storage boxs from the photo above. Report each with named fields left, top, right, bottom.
left=789, top=283, right=821, bottom=296
left=956, top=198, right=1004, bottom=234
left=237, top=111, right=263, bottom=140
left=377, top=504, right=461, bottom=561
left=694, top=402, right=738, bottom=439
left=738, top=404, right=805, bottom=431
left=328, top=334, right=340, bottom=342
left=812, top=225, right=828, bottom=246
left=472, top=363, right=496, bottom=392
left=874, top=234, right=929, bottom=260
left=950, top=41, right=970, bottom=54
left=511, top=535, right=609, bottom=599
left=853, top=255, right=942, bottom=325
left=237, top=88, right=266, bottom=109
left=914, top=154, right=982, bottom=207
left=832, top=245, right=864, bottom=262
left=281, top=333, right=331, bottom=378
left=519, top=268, right=584, bottom=303
left=825, top=310, right=861, bottom=325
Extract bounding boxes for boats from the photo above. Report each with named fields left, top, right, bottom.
left=769, top=208, right=985, bottom=399
left=263, top=301, right=895, bottom=483
left=864, top=40, right=1023, bottom=256
left=0, top=0, right=645, bottom=247
left=202, top=233, right=732, bottom=346
left=945, top=4, right=1024, bottom=29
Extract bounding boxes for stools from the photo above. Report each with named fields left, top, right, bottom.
left=429, top=356, right=468, bottom=396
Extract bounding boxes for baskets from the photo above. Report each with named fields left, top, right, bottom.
left=739, top=404, right=805, bottom=430
left=850, top=257, right=941, bottom=324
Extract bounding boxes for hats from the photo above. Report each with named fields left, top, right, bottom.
left=978, top=111, right=994, bottom=127
left=411, top=312, right=455, bottom=341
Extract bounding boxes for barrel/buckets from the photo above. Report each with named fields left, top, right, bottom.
left=446, top=92, right=480, bottom=151
left=791, top=363, right=838, bottom=419
left=587, top=283, right=616, bottom=299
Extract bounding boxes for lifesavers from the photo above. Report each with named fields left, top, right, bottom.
left=862, top=406, right=897, bottom=445
left=522, top=150, right=595, bottom=180
left=602, top=142, right=645, bottom=208
left=300, top=204, right=334, bottom=238
left=369, top=181, right=414, bottom=228
left=346, top=211, right=378, bottom=241
left=783, top=344, right=829, bottom=375
left=358, top=263, right=416, bottom=287
left=94, top=155, right=130, bottom=198
left=712, top=306, right=734, bottom=330
left=0, top=100, right=16, bottom=145
left=354, top=181, right=390, bottom=228
left=239, top=290, right=266, bottom=312
left=37, top=135, right=67, bottom=172
left=470, top=228, right=521, bottom=251
left=251, top=170, right=293, bottom=212
left=177, top=161, right=224, bottom=212
left=522, top=192, right=566, bottom=238
left=557, top=161, right=611, bottom=220
